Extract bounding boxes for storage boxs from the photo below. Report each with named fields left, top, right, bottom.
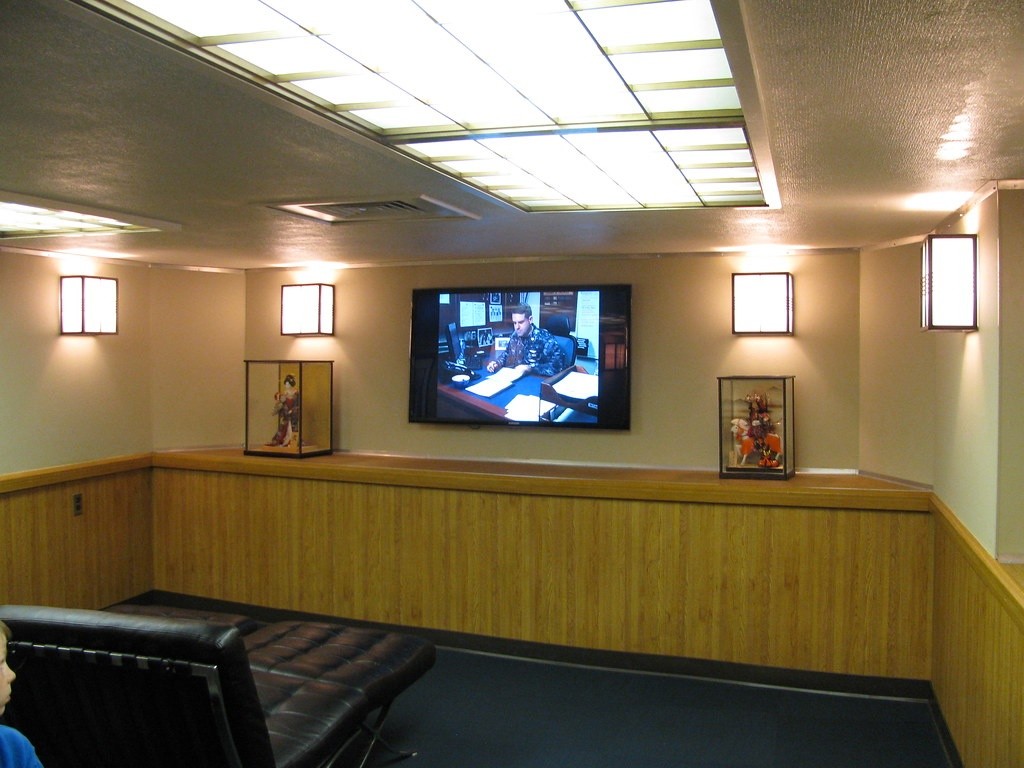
left=241, top=359, right=335, bottom=460
left=716, top=374, right=796, bottom=480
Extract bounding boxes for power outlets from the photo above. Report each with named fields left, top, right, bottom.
left=73, top=494, right=83, bottom=516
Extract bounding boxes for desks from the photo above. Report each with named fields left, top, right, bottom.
left=438, top=358, right=552, bottom=421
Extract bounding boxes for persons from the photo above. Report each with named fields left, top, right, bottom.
left=487, top=301, right=564, bottom=376
left=265, top=374, right=301, bottom=446
left=483, top=330, right=489, bottom=342
left=0, top=622, right=47, bottom=768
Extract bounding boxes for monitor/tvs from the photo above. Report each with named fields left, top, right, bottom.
left=408, top=283, right=632, bottom=430
left=444, top=322, right=462, bottom=363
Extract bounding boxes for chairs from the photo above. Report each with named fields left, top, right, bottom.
left=542, top=314, right=575, bottom=368
left=1, top=601, right=439, bottom=767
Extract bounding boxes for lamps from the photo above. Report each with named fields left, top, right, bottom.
left=280, top=283, right=336, bottom=336
left=919, top=233, right=981, bottom=332
left=730, top=273, right=794, bottom=336
left=58, top=274, right=118, bottom=334
